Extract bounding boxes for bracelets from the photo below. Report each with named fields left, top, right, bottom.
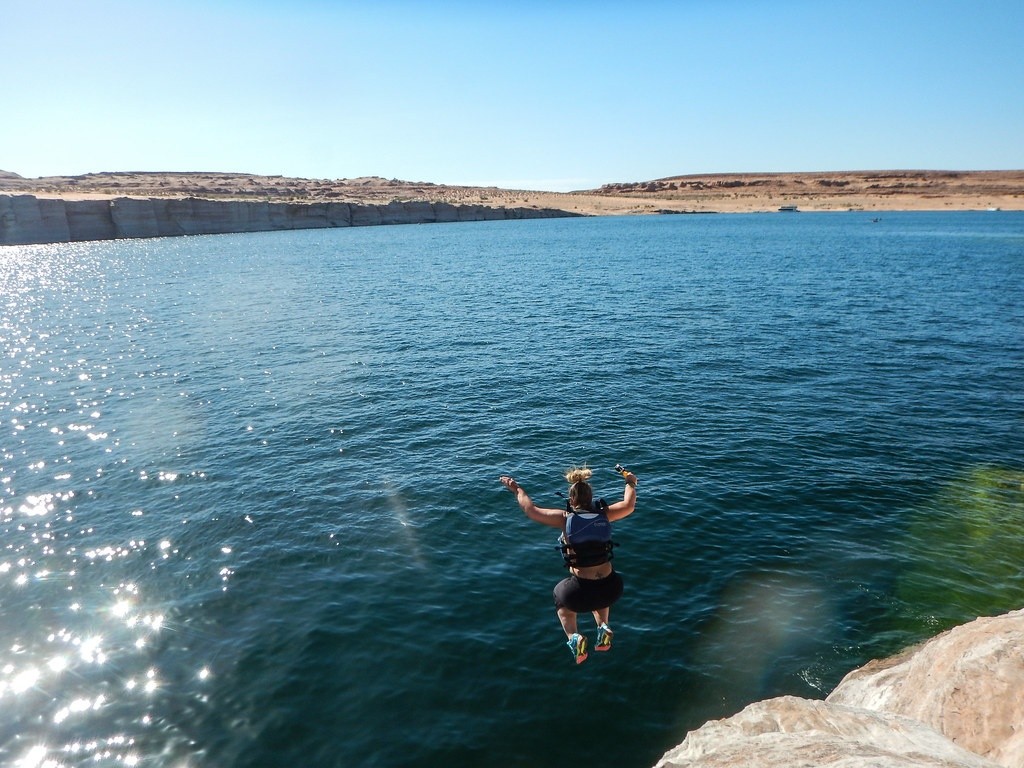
left=625, top=482, right=635, bottom=488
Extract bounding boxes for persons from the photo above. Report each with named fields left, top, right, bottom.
left=500, top=469, right=638, bottom=664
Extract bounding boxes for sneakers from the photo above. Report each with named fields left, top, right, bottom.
left=594, top=622, right=613, bottom=651
left=567, top=632, right=588, bottom=663
